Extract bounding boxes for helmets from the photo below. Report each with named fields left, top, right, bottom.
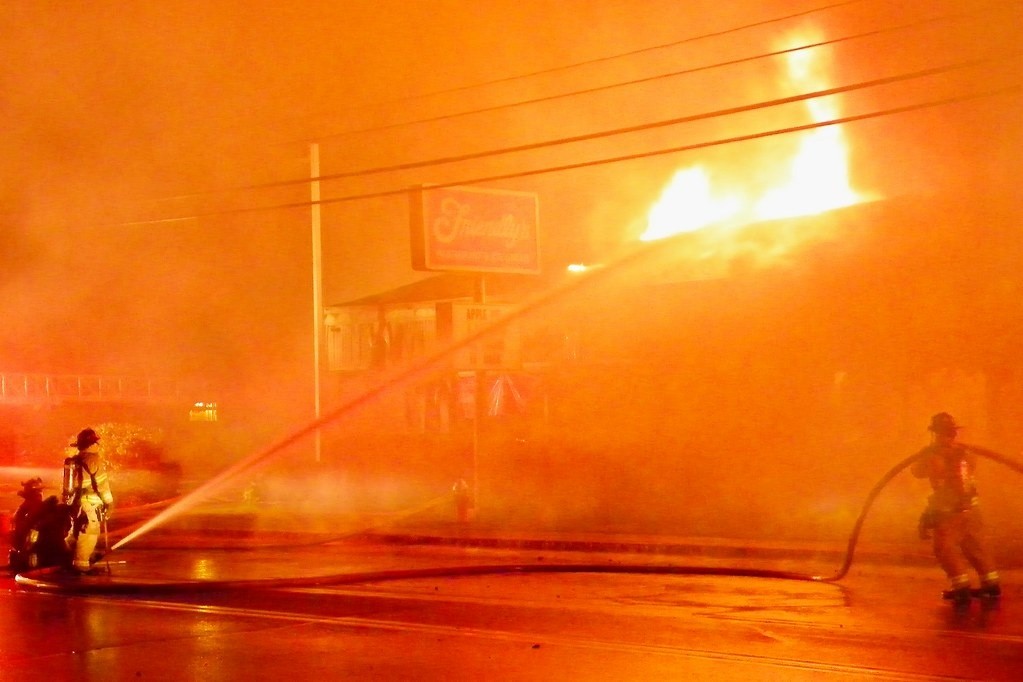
left=78, top=427, right=100, bottom=451
left=927, top=412, right=958, bottom=431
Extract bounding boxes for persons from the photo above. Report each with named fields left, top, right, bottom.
left=910, top=412, right=1002, bottom=601
left=64, top=430, right=114, bottom=575
left=453, top=476, right=471, bottom=524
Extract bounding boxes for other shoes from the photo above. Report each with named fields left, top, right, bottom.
left=973, top=587, right=999, bottom=598
left=943, top=587, right=970, bottom=598
left=72, top=563, right=106, bottom=575
